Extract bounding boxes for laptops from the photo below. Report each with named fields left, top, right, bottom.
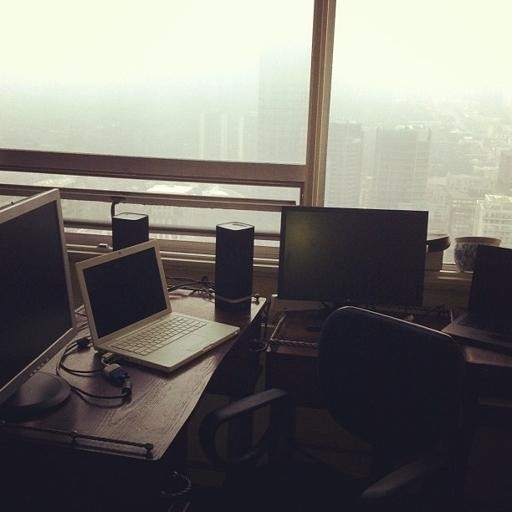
left=441, top=244, right=512, bottom=354
left=75, top=240, right=241, bottom=373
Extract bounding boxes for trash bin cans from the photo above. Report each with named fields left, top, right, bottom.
left=159, top=474, right=192, bottom=512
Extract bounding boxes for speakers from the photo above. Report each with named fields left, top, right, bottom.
left=112, top=212, right=149, bottom=251
left=215, top=221, right=254, bottom=312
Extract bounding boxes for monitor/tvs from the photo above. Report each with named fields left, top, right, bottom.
left=278, top=207, right=428, bottom=332
left=0, top=188, right=79, bottom=423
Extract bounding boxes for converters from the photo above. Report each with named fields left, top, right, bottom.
left=103, top=363, right=132, bottom=396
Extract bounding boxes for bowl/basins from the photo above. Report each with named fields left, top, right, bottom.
left=453, top=236, right=500, bottom=271
